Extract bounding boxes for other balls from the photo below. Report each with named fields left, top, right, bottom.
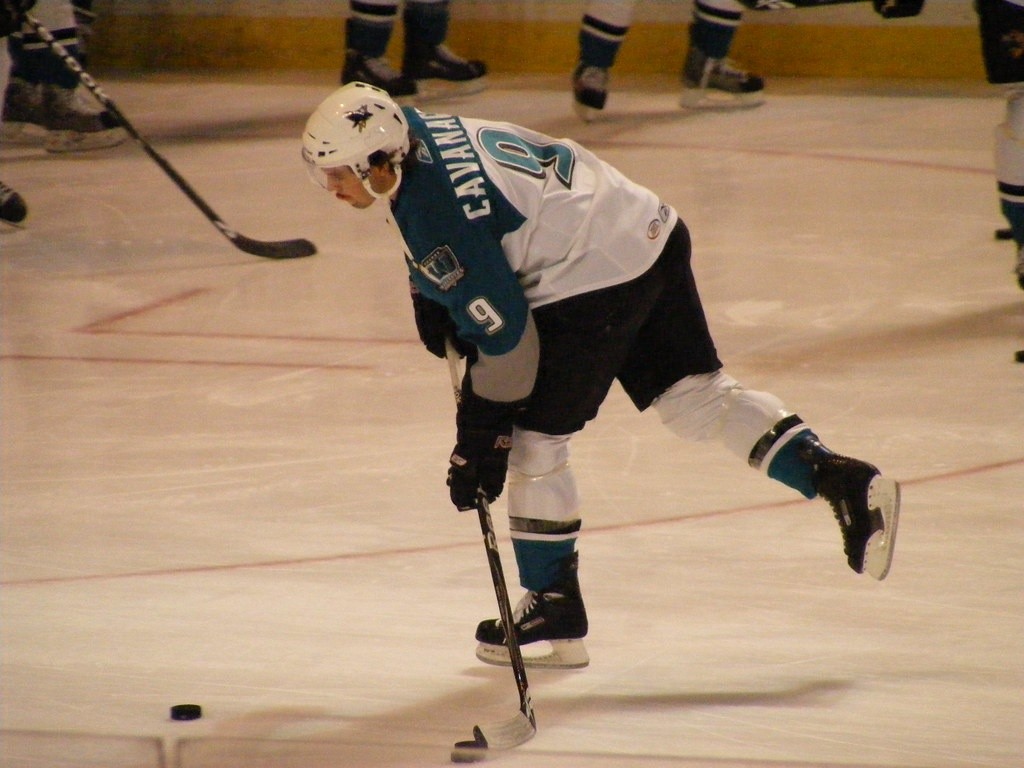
left=450, top=740, right=486, bottom=764
left=169, top=703, right=202, bottom=721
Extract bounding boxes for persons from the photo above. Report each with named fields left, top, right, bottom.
left=973, top=1, right=1024, bottom=290
left=0, top=0, right=129, bottom=230
left=300, top=81, right=900, bottom=668
left=342, top=0, right=485, bottom=94
left=572, top=0, right=766, bottom=121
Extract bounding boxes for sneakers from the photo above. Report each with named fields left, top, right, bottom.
left=0, top=181, right=28, bottom=229
left=46, top=94, right=131, bottom=153
left=678, top=53, right=766, bottom=110
left=572, top=62, right=607, bottom=122
left=475, top=553, right=592, bottom=669
left=341, top=54, right=420, bottom=109
left=0, top=82, right=58, bottom=144
left=400, top=45, right=488, bottom=100
left=801, top=436, right=899, bottom=581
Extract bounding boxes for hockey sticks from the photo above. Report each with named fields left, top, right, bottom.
left=736, top=0, right=869, bottom=14
left=443, top=338, right=538, bottom=752
left=7, top=3, right=319, bottom=261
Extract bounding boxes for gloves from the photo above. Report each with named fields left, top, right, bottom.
left=447, top=406, right=515, bottom=511
left=408, top=275, right=476, bottom=359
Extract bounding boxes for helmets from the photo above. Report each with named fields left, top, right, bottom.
left=302, top=82, right=409, bottom=199
